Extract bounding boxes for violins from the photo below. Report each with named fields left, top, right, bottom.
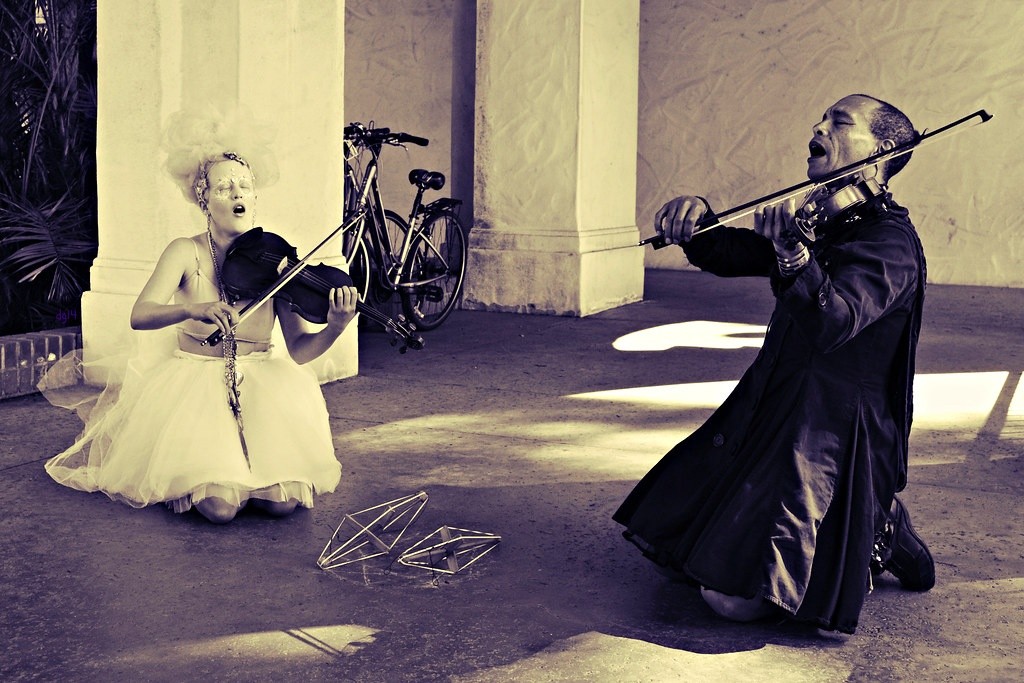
left=222, top=227, right=425, bottom=355
left=782, top=177, right=886, bottom=252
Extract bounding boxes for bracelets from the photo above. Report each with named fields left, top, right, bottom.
left=761, top=225, right=815, bottom=279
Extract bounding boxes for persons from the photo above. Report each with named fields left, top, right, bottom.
left=36, top=89, right=362, bottom=521
left=612, top=95, right=937, bottom=630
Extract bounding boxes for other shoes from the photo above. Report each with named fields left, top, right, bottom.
left=870, top=494, right=935, bottom=593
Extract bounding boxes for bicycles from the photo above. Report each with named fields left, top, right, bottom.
left=342, top=119, right=469, bottom=333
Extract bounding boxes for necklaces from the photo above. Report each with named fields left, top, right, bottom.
left=204, top=228, right=251, bottom=468
